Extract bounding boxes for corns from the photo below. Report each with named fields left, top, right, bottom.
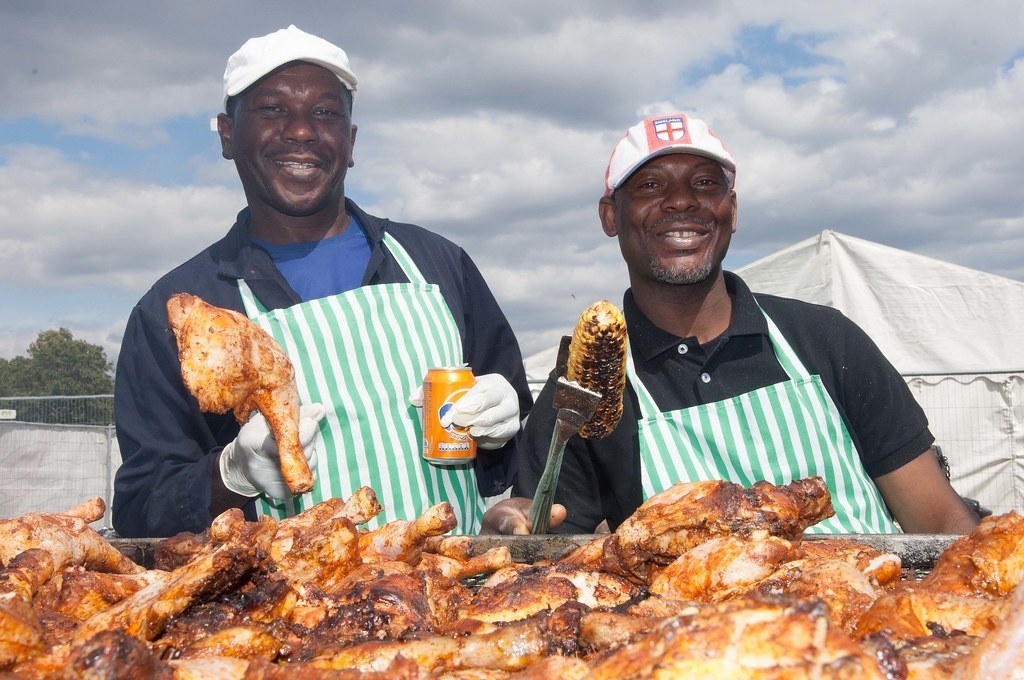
left=567, top=300, right=628, bottom=439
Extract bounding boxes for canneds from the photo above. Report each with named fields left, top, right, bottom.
left=421, top=362, right=478, bottom=465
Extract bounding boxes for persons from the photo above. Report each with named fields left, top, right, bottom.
left=477, top=114, right=982, bottom=536
left=112, top=25, right=534, bottom=539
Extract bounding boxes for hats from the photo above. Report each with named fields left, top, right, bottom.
left=210, top=23, right=360, bottom=130
left=602, top=111, right=738, bottom=197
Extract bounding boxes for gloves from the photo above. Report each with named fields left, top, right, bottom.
left=219, top=402, right=326, bottom=500
left=408, top=373, right=520, bottom=450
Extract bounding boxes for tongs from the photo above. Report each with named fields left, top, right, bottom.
left=523, top=373, right=598, bottom=533
